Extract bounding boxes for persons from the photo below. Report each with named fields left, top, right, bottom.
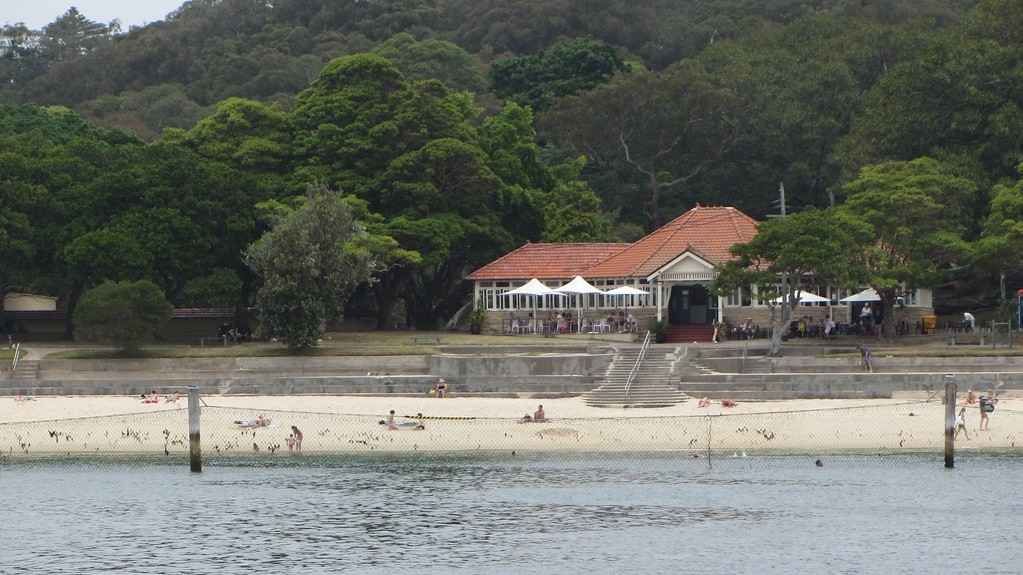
left=287, top=425, right=302, bottom=452
left=385, top=409, right=400, bottom=430
left=979, top=395, right=990, bottom=431
left=436, top=378, right=447, bottom=398
left=954, top=406, right=973, bottom=440
left=790, top=315, right=830, bottom=339
left=852, top=302, right=976, bottom=340
left=856, top=346, right=873, bottom=373
left=507, top=311, right=631, bottom=333
left=965, top=390, right=976, bottom=405
left=233, top=415, right=272, bottom=429
left=987, top=389, right=998, bottom=405
left=738, top=317, right=757, bottom=340
left=139, top=391, right=180, bottom=402
left=815, top=459, right=823, bottom=466
left=533, top=405, right=544, bottom=419
left=410, top=413, right=425, bottom=430
left=698, top=396, right=738, bottom=407
left=512, top=451, right=517, bottom=456
left=231, top=327, right=277, bottom=342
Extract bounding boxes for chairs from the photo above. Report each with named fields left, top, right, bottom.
left=502, top=315, right=639, bottom=335
left=895, top=319, right=992, bottom=335
left=730, top=323, right=879, bottom=339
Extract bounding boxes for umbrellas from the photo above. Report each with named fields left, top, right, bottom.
left=838, top=287, right=903, bottom=335
left=768, top=290, right=832, bottom=310
left=544, top=275, right=605, bottom=334
left=600, top=286, right=651, bottom=333
left=497, top=278, right=571, bottom=334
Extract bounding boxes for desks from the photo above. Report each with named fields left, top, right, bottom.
left=924, top=327, right=936, bottom=334
left=567, top=321, right=573, bottom=333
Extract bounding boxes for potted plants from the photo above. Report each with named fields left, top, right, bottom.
left=647, top=320, right=672, bottom=343
left=469, top=298, right=488, bottom=334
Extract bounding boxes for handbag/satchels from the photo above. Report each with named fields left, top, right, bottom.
left=430, top=389, right=436, bottom=394
left=958, top=412, right=964, bottom=425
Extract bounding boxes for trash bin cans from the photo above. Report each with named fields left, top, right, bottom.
left=921, top=316, right=937, bottom=334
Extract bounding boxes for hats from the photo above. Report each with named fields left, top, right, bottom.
left=747, top=317, right=752, bottom=319
left=439, top=379, right=444, bottom=382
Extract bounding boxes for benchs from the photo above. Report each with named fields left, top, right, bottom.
left=199, top=337, right=231, bottom=345
